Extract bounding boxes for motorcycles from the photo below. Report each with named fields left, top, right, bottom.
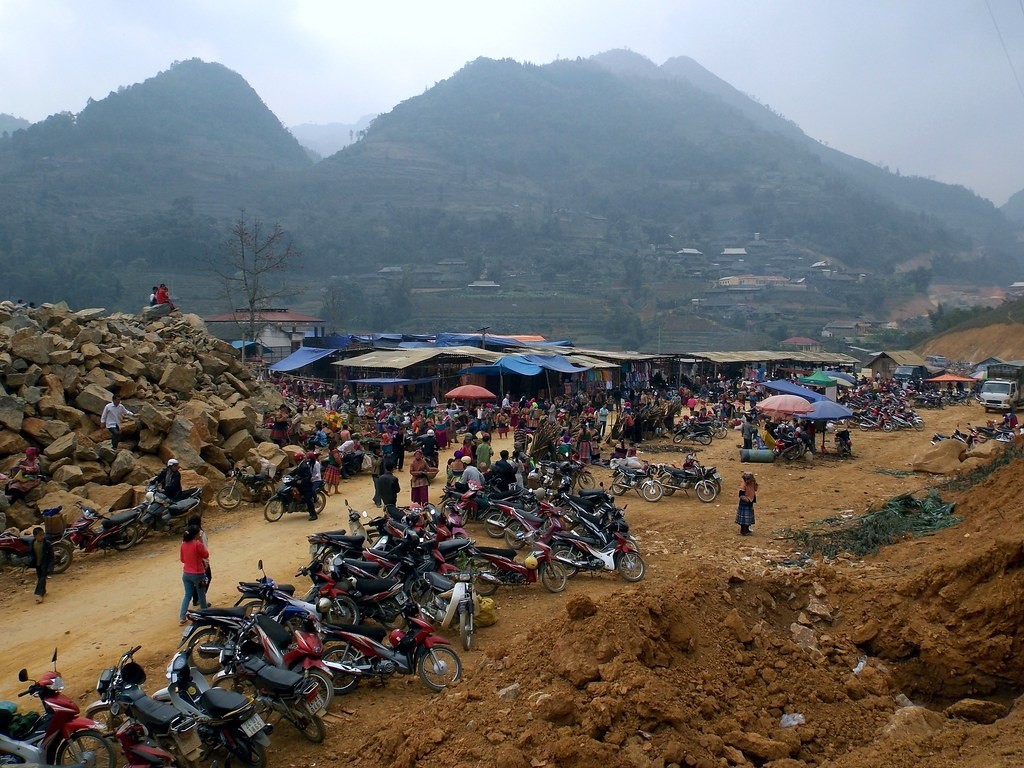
left=216, top=464, right=275, bottom=511
left=0, top=380, right=810, bottom=768
left=835, top=429, right=853, bottom=456
left=262, top=473, right=330, bottom=522
left=0, top=526, right=79, bottom=575
left=64, top=501, right=144, bottom=552
left=840, top=383, right=971, bottom=433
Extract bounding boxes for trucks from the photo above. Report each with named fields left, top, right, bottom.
left=891, top=363, right=945, bottom=391
left=978, top=360, right=1024, bottom=414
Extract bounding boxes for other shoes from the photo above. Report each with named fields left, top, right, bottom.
left=373, top=497, right=375, bottom=501
left=308, top=515, right=318, bottom=521
left=179, top=619, right=189, bottom=625
left=44, top=592, right=48, bottom=596
left=36, top=597, right=43, bottom=603
left=377, top=505, right=381, bottom=508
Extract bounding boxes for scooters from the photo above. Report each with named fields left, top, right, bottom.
left=929, top=412, right=1016, bottom=448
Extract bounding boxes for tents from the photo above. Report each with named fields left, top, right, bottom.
left=798, top=369, right=837, bottom=386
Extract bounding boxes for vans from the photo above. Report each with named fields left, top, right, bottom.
left=926, top=355, right=947, bottom=368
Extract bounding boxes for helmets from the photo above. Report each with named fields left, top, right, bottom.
left=389, top=629, right=406, bottom=647
left=427, top=429, right=434, bottom=436
left=306, top=452, right=314, bottom=458
left=465, top=433, right=472, bottom=440
left=536, top=488, right=545, bottom=500
left=167, top=459, right=178, bottom=468
left=294, top=453, right=304, bottom=462
left=524, top=556, right=538, bottom=569
left=120, top=661, right=146, bottom=685
left=461, top=456, right=471, bottom=464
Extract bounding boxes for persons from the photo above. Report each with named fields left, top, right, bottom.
left=956, top=382, right=963, bottom=389
left=17, top=299, right=34, bottom=308
left=947, top=382, right=953, bottom=389
left=179, top=515, right=212, bottom=625
left=5, top=446, right=40, bottom=507
left=678, top=373, right=766, bottom=418
left=876, top=371, right=880, bottom=378
left=735, top=472, right=758, bottom=534
left=28, top=527, right=55, bottom=604
left=262, top=376, right=673, bottom=521
left=144, top=458, right=182, bottom=498
left=764, top=418, right=814, bottom=450
left=101, top=394, right=140, bottom=449
left=150, top=284, right=176, bottom=311
left=862, top=375, right=867, bottom=381
left=902, top=379, right=914, bottom=390
left=741, top=417, right=756, bottom=449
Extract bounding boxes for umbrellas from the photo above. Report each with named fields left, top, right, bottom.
left=793, top=401, right=853, bottom=446
left=756, top=394, right=816, bottom=428
left=444, top=385, right=496, bottom=399
